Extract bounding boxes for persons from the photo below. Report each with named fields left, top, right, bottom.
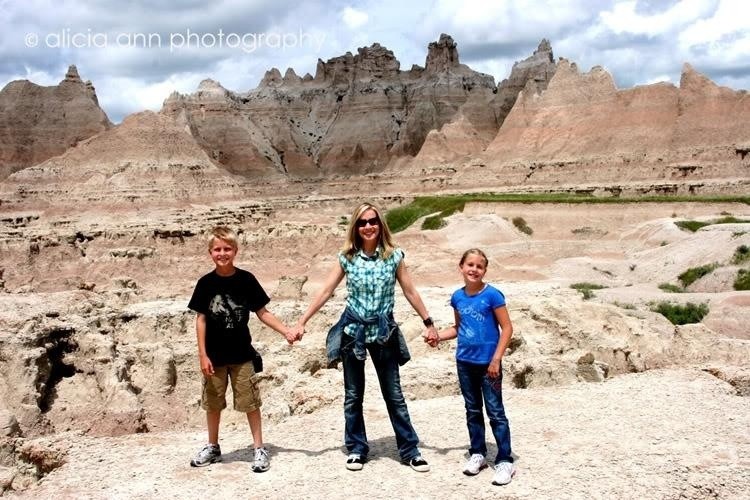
left=186, top=227, right=296, bottom=473
left=423, top=249, right=520, bottom=487
left=294, top=202, right=440, bottom=472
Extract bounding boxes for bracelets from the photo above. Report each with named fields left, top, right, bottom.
left=424, top=317, right=433, bottom=326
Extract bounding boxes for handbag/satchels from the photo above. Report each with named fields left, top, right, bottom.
left=253, top=352, right=264, bottom=373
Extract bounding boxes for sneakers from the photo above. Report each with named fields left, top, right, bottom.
left=345, top=453, right=364, bottom=471
left=462, top=454, right=489, bottom=476
left=188, top=444, right=223, bottom=468
left=491, top=461, right=516, bottom=486
left=404, top=454, right=429, bottom=473
left=251, top=448, right=271, bottom=472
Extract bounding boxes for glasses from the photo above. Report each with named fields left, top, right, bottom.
left=357, top=218, right=379, bottom=227
